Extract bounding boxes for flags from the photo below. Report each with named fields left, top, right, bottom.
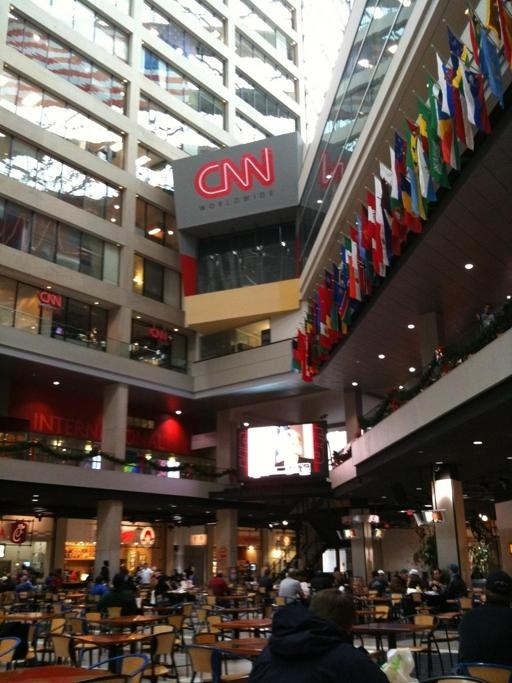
left=292, top=0, right=512, bottom=383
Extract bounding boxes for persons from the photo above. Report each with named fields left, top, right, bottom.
left=458, top=572, right=510, bottom=662
left=248, top=587, right=389, bottom=681
left=2, top=558, right=466, bottom=618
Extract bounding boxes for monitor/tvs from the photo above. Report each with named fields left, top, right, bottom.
left=336, top=515, right=384, bottom=540
left=237, top=421, right=328, bottom=482
left=249, top=563, right=257, bottom=571
left=0, top=544, right=5, bottom=557
left=413, top=509, right=447, bottom=527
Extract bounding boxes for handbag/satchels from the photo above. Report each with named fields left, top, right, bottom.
left=380, top=648, right=419, bottom=683
